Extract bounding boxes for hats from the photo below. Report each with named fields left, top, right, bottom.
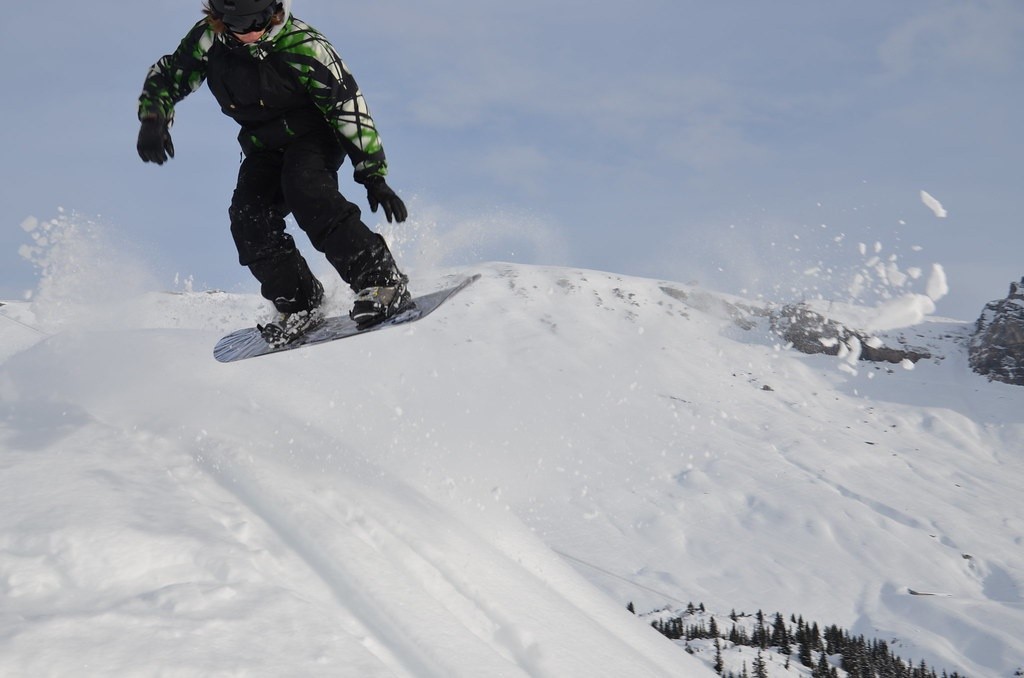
left=211, top=0, right=273, bottom=15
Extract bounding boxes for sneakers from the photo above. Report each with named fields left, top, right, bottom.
left=349, top=274, right=408, bottom=327
left=261, top=305, right=325, bottom=348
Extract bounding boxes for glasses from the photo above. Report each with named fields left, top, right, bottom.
left=221, top=7, right=273, bottom=35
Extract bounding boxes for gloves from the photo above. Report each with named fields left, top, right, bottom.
left=136, top=118, right=174, bottom=165
left=364, top=175, right=408, bottom=223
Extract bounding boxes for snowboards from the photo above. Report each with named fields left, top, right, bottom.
left=213, top=271, right=485, bottom=362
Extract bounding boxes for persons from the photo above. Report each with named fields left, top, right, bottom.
left=137, top=0, right=422, bottom=348
